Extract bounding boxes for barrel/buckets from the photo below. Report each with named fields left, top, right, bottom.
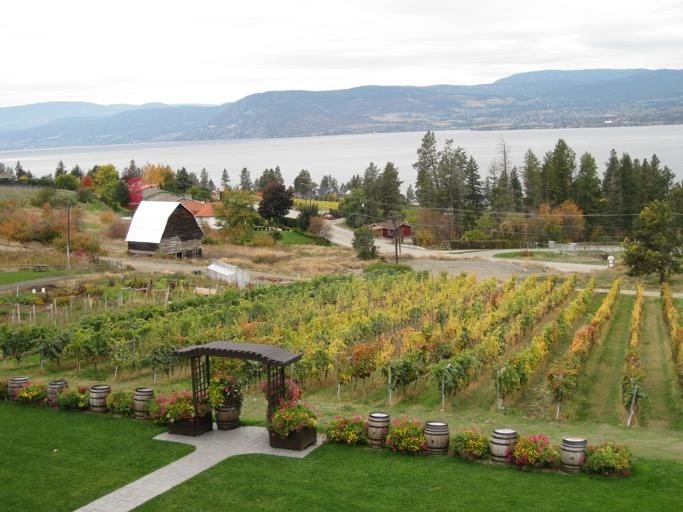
left=368, top=412, right=390, bottom=448
left=215, top=400, right=238, bottom=430
left=8, top=376, right=29, bottom=400
left=47, top=381, right=68, bottom=407
left=490, top=429, right=516, bottom=465
left=424, top=421, right=449, bottom=456
left=90, top=385, right=111, bottom=413
left=133, top=388, right=154, bottom=420
left=560, top=436, right=587, bottom=473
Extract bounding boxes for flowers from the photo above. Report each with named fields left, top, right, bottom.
left=259, top=376, right=320, bottom=439
left=143, top=390, right=211, bottom=425
left=207, top=367, right=245, bottom=407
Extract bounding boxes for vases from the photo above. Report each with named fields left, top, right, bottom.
left=214, top=405, right=239, bottom=430
